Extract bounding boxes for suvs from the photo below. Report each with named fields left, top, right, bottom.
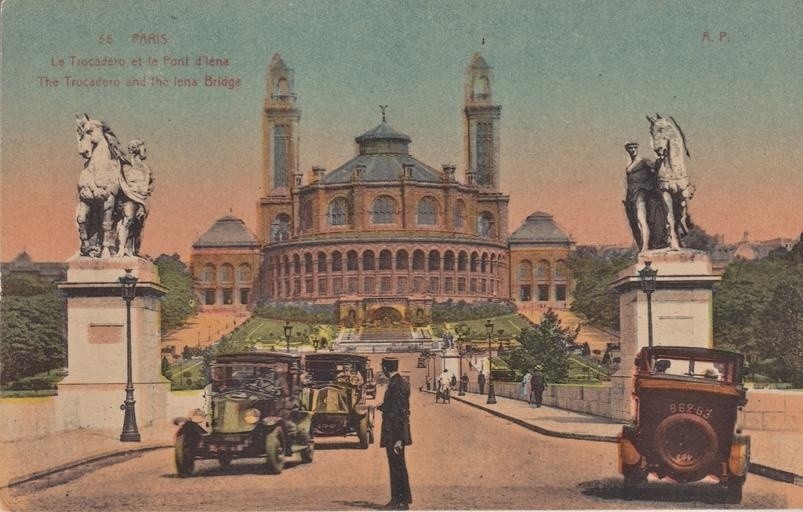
left=173, top=352, right=377, bottom=472
left=615, top=346, right=752, bottom=503
left=159, top=344, right=175, bottom=354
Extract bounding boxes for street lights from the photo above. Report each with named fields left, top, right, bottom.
left=484, top=320, right=493, bottom=377
left=282, top=318, right=292, bottom=352
left=117, top=266, right=138, bottom=389
left=636, top=260, right=659, bottom=346
left=425, top=334, right=463, bottom=378
left=311, top=335, right=320, bottom=353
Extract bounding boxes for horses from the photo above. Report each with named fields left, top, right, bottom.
left=646, top=112, right=691, bottom=253
left=75, top=112, right=144, bottom=259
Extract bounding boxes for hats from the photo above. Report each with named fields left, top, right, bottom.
left=535, top=364, right=544, bottom=370
left=382, top=356, right=398, bottom=371
left=705, top=368, right=718, bottom=377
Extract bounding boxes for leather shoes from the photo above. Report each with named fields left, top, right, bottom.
left=379, top=499, right=412, bottom=510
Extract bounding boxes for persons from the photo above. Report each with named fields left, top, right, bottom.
left=252, top=367, right=289, bottom=397
left=114, top=136, right=153, bottom=262
left=522, top=368, right=534, bottom=406
left=477, top=370, right=485, bottom=395
left=530, top=364, right=549, bottom=408
left=336, top=363, right=364, bottom=387
left=451, top=373, right=457, bottom=391
left=655, top=360, right=671, bottom=374
left=617, top=139, right=663, bottom=252
left=436, top=369, right=453, bottom=394
left=461, top=373, right=469, bottom=392
left=371, top=356, right=413, bottom=510
left=704, top=368, right=721, bottom=381
